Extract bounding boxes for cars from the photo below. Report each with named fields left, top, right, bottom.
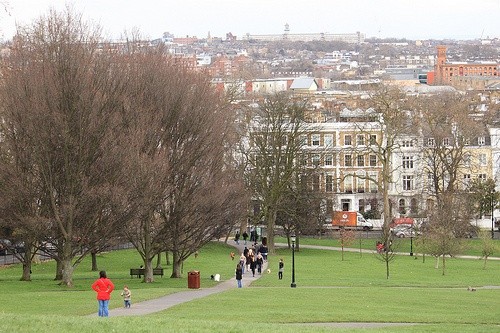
left=0, top=236, right=65, bottom=256
left=380, top=216, right=428, bottom=239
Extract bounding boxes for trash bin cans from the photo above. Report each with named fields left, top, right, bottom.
left=376, top=240, right=382, bottom=246
left=188, top=270, right=200, bottom=289
left=262, top=236, right=268, bottom=245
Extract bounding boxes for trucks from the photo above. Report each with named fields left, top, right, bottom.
left=331, top=210, right=373, bottom=232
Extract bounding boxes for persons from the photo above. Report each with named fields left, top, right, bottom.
left=278, top=258, right=284, bottom=280
left=195, top=250, right=198, bottom=258
left=120, top=285, right=131, bottom=308
left=230, top=251, right=235, bottom=261
left=239, top=241, right=269, bottom=277
left=250, top=228, right=258, bottom=241
left=242, top=231, right=248, bottom=245
left=376, top=238, right=393, bottom=255
left=235, top=231, right=240, bottom=245
left=235, top=264, right=243, bottom=289
left=92, top=270, right=114, bottom=317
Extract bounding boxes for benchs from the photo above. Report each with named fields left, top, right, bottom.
left=130, top=269, right=163, bottom=278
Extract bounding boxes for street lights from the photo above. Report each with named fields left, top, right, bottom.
left=408, top=227, right=413, bottom=256
left=290, top=231, right=297, bottom=288
left=490, top=191, right=494, bottom=239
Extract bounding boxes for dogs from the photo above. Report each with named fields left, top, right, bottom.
left=210, top=274, right=220, bottom=281
left=267, top=269, right=271, bottom=275
